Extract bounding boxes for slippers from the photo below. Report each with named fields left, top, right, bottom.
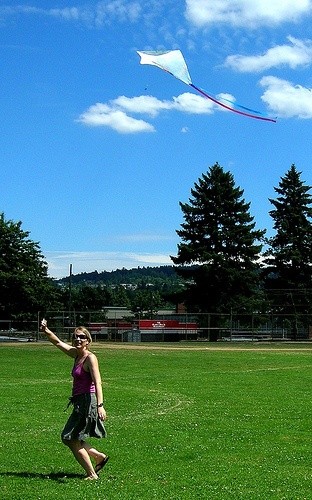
left=95, top=456, right=109, bottom=473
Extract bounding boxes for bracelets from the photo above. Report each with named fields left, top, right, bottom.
left=97, top=403, right=103, bottom=407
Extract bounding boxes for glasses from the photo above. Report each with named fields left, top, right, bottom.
left=73, top=334, right=89, bottom=340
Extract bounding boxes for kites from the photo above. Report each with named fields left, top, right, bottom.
left=136, top=50, right=278, bottom=123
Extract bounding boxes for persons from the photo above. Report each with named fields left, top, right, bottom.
left=41, top=320, right=110, bottom=480
